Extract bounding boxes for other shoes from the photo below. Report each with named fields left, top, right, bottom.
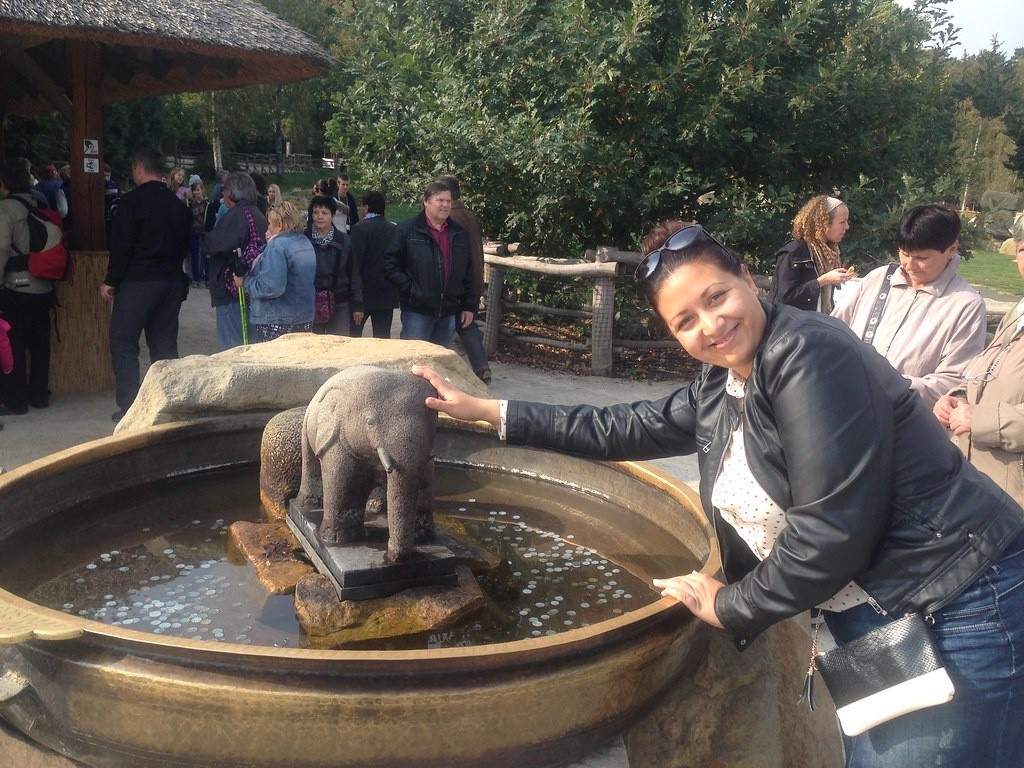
left=192, top=281, right=200, bottom=288
left=112, top=409, right=127, bottom=422
left=205, top=280, right=210, bottom=289
left=476, top=367, right=491, bottom=385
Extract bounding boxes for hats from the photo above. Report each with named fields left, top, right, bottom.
left=43, top=166, right=57, bottom=180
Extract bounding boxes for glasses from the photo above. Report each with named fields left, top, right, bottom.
left=634, top=224, right=732, bottom=283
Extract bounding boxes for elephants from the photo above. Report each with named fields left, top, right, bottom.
left=297, top=355, right=441, bottom=565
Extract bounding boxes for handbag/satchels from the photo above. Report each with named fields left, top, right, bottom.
left=314, top=290, right=334, bottom=325
left=221, top=203, right=266, bottom=299
left=796, top=613, right=956, bottom=737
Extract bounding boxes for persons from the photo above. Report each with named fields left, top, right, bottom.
left=33, top=166, right=68, bottom=220
left=413, top=237, right=1023, bottom=768
left=17, top=158, right=35, bottom=184
left=0, top=319, right=14, bottom=431
left=0, top=168, right=56, bottom=414
left=267, top=185, right=281, bottom=206
left=200, top=173, right=269, bottom=352
left=232, top=201, right=316, bottom=343
left=434, top=175, right=492, bottom=383
left=99, top=148, right=193, bottom=422
left=102, top=163, right=230, bottom=288
left=934, top=212, right=1024, bottom=511
left=304, top=195, right=364, bottom=336
left=350, top=190, right=398, bottom=339
left=57, top=166, right=70, bottom=199
left=337, top=173, right=359, bottom=233
left=400, top=183, right=479, bottom=346
left=832, top=205, right=986, bottom=440
left=250, top=171, right=268, bottom=215
left=313, top=179, right=351, bottom=232
left=766, top=195, right=855, bottom=315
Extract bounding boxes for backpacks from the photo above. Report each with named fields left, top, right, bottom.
left=0, top=195, right=75, bottom=286
